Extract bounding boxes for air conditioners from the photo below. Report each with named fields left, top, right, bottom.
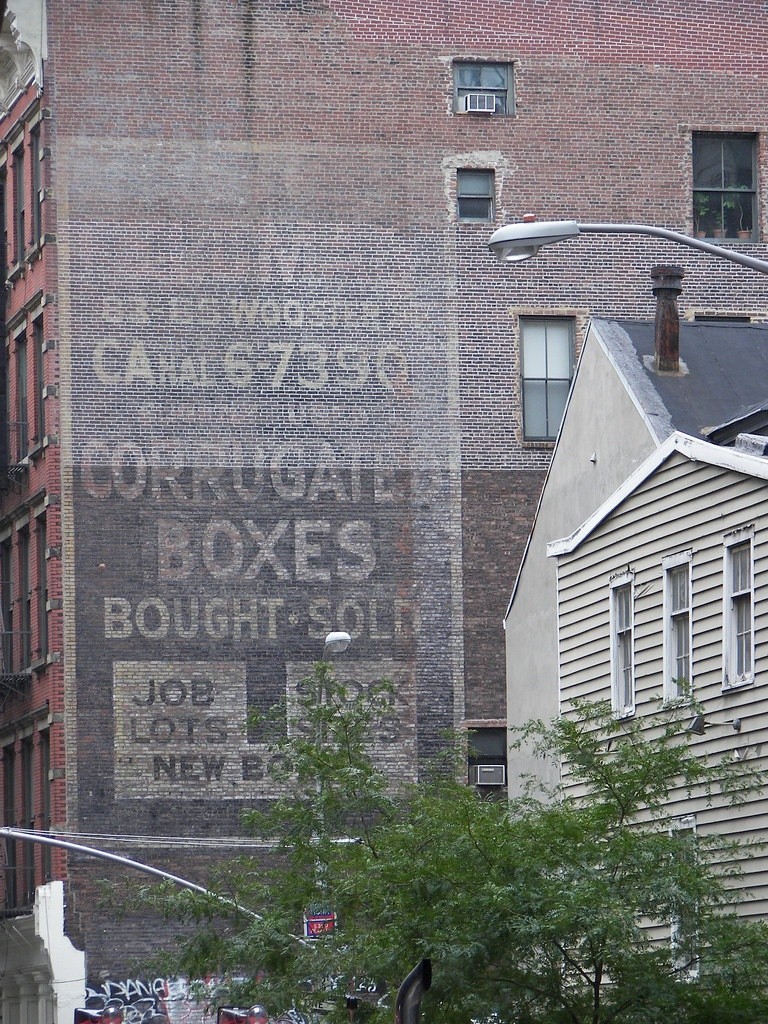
left=473, top=764, right=506, bottom=786
left=464, top=93, right=496, bottom=114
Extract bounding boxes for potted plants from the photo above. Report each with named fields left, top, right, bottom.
left=711, top=210, right=728, bottom=238
left=693, top=191, right=709, bottom=238
left=723, top=183, right=752, bottom=239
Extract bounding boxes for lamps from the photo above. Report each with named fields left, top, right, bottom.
left=684, top=716, right=741, bottom=736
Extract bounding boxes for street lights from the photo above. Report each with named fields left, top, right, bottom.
left=312, top=629, right=352, bottom=892
